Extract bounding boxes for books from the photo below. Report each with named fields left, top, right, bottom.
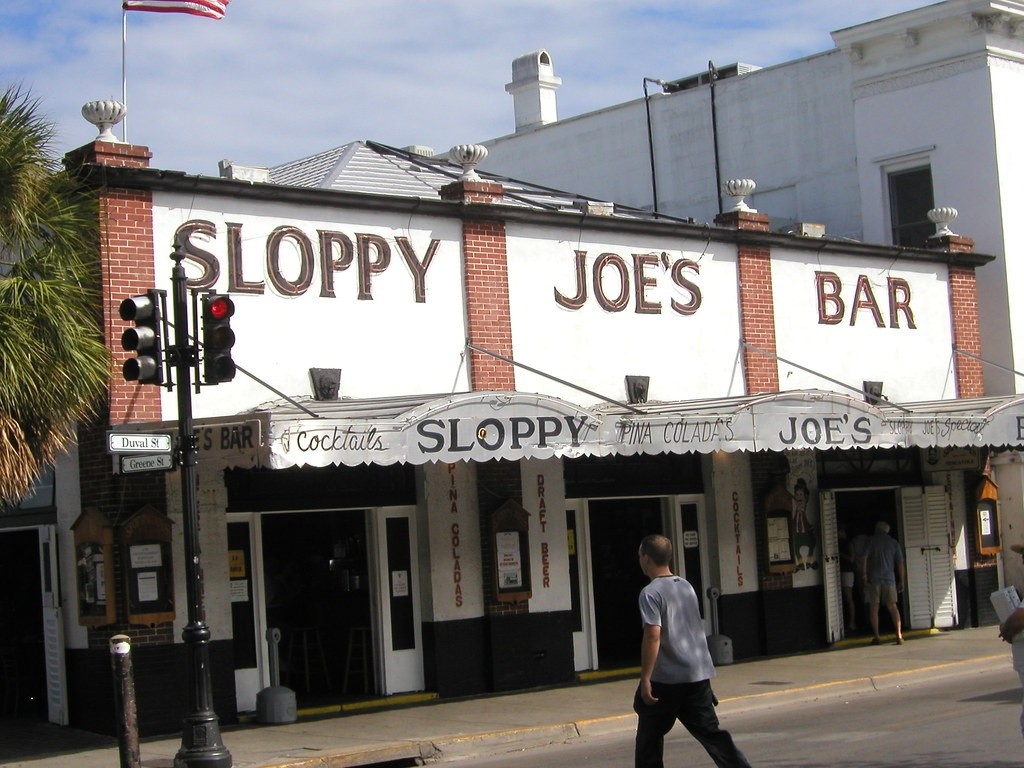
left=989, top=585, right=1024, bottom=643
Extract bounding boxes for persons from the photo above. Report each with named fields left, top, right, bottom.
left=633, top=534, right=750, bottom=768
left=862, top=521, right=905, bottom=644
left=838, top=526, right=862, bottom=638
left=1000, top=546, right=1024, bottom=738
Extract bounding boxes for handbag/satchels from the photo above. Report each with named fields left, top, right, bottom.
left=852, top=576, right=864, bottom=601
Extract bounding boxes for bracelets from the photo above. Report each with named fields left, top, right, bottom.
left=861, top=574, right=867, bottom=580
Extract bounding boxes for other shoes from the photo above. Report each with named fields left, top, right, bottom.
left=896, top=639, right=904, bottom=645
left=847, top=625, right=858, bottom=632
left=867, top=639, right=881, bottom=646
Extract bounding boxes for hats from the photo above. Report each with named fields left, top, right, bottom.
left=1010, top=544, right=1024, bottom=554
left=872, top=521, right=890, bottom=533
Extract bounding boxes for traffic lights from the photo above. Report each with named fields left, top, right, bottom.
left=201, top=293, right=236, bottom=384
left=119, top=288, right=162, bottom=386
